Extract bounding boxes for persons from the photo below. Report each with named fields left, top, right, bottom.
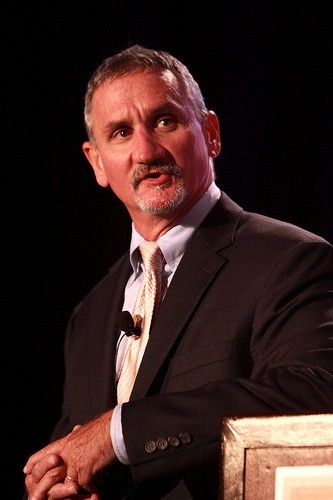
left=22, top=45, right=332, bottom=500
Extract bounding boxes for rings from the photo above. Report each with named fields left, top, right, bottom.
left=66, top=476, right=78, bottom=482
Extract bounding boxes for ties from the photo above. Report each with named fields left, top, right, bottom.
left=117, top=240, right=164, bottom=409
